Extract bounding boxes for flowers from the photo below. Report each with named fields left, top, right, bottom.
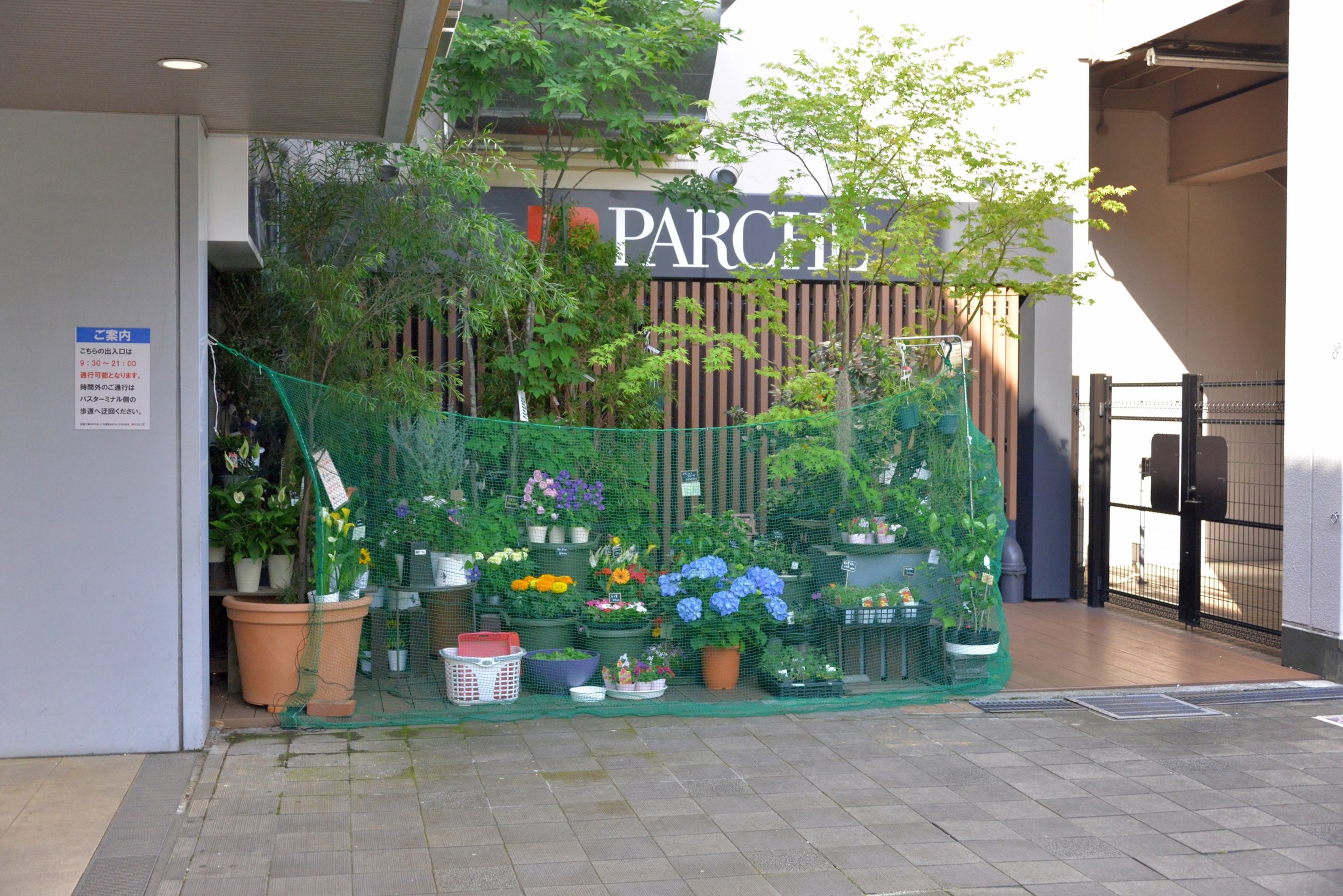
left=209, top=327, right=1010, bottom=683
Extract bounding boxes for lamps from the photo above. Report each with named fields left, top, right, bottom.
left=709, top=162, right=743, bottom=190
left=374, top=152, right=399, bottom=181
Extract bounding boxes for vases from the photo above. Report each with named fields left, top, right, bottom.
left=529, top=540, right=592, bottom=594
left=700, top=645, right=740, bottom=690
left=434, top=557, right=467, bottom=587
left=388, top=649, right=408, bottom=672
left=408, top=591, right=422, bottom=607
left=569, top=526, right=590, bottom=543
left=364, top=587, right=384, bottom=608
left=385, top=587, right=408, bottom=610
left=548, top=524, right=566, bottom=544
left=430, top=551, right=445, bottom=582
left=222, top=595, right=378, bottom=707
left=778, top=573, right=813, bottom=612
left=267, top=554, right=295, bottom=589
left=233, top=558, right=263, bottom=593
left=526, top=526, right=548, bottom=543
left=501, top=613, right=579, bottom=652
left=574, top=618, right=653, bottom=682
left=449, top=553, right=474, bottom=584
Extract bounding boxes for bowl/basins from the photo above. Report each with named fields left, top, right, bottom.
left=569, top=686, right=607, bottom=703
